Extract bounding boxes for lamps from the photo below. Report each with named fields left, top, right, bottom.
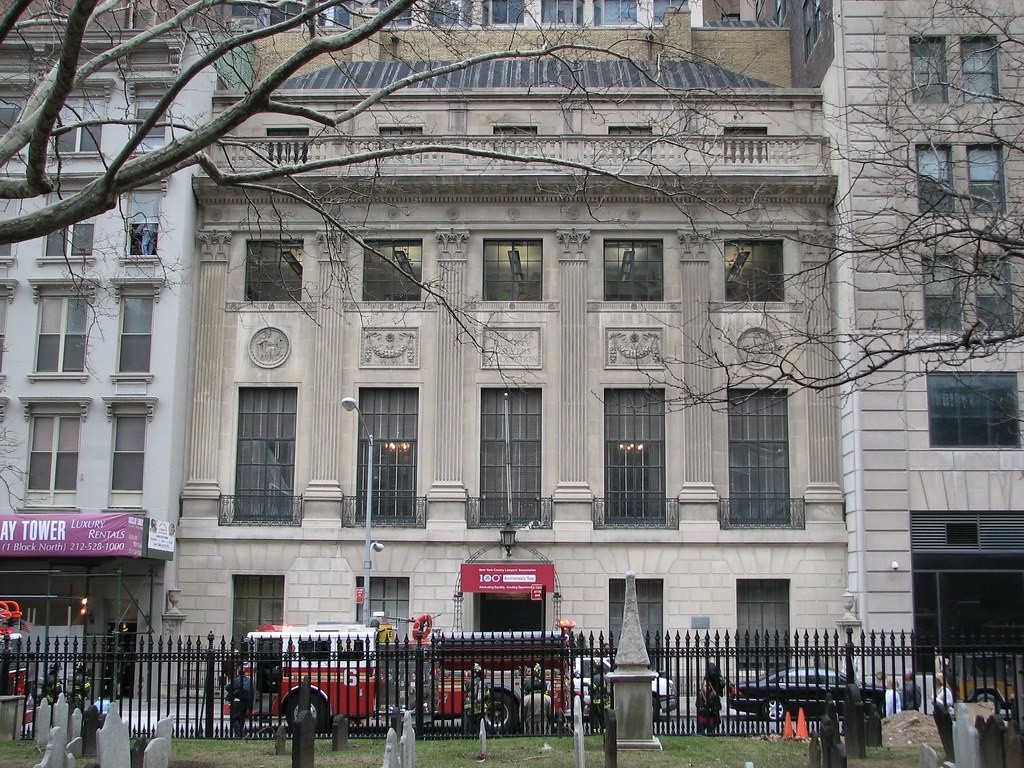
left=499, top=521, right=517, bottom=557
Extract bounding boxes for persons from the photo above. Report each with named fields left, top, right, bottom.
left=42, top=647, right=951, bottom=738
left=132, top=223, right=158, bottom=255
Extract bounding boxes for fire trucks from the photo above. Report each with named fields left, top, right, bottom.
left=0, top=600, right=38, bottom=725
left=224, top=614, right=573, bottom=736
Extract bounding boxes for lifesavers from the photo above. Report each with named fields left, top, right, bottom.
left=412, top=613, right=433, bottom=640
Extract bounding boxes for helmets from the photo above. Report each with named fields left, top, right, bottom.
left=75, top=660, right=83, bottom=670
left=589, top=659, right=602, bottom=670
left=49, top=661, right=65, bottom=670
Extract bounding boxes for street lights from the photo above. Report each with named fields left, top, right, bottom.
left=340, top=396, right=384, bottom=627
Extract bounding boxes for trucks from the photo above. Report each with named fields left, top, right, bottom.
left=949, top=652, right=1015, bottom=717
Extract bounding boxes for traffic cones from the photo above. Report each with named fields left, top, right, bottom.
left=795, top=707, right=810, bottom=742
left=783, top=711, right=795, bottom=739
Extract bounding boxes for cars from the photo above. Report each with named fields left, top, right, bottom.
left=568, top=654, right=681, bottom=734
left=728, top=666, right=905, bottom=723
